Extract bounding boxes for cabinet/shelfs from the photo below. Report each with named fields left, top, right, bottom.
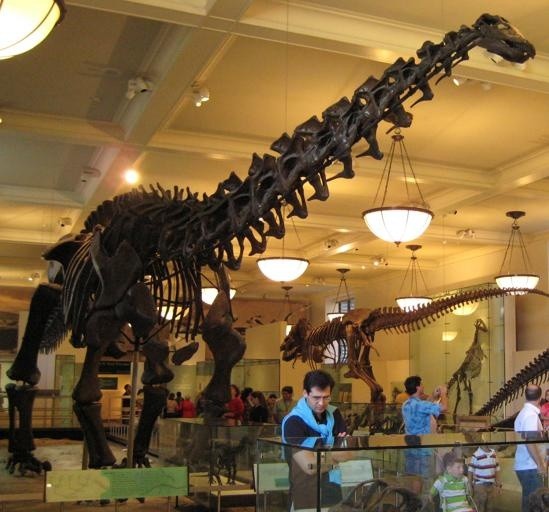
left=257, top=431, right=549, bottom=512
left=326, top=400, right=405, bottom=434
left=148, top=416, right=282, bottom=508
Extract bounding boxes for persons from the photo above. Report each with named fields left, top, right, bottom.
left=121, top=383, right=131, bottom=424
left=248, top=391, right=268, bottom=423
left=282, top=370, right=355, bottom=512
left=241, top=387, right=253, bottom=423
left=402, top=375, right=450, bottom=435
left=403, top=434, right=442, bottom=477
left=427, top=451, right=478, bottom=512
left=176, top=393, right=194, bottom=418
left=541, top=389, right=549, bottom=430
left=468, top=428, right=504, bottom=512
left=223, top=384, right=245, bottom=427
left=166, top=393, right=178, bottom=418
left=276, top=386, right=297, bottom=424
left=174, top=391, right=185, bottom=418
left=513, top=384, right=548, bottom=511
left=267, top=394, right=277, bottom=425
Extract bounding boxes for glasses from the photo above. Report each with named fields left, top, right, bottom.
left=311, top=397, right=332, bottom=402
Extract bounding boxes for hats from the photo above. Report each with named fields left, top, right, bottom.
left=282, top=386, right=293, bottom=394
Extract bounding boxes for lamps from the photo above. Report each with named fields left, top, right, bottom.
left=194, top=86, right=211, bottom=108
left=362, top=134, right=434, bottom=246
left=395, top=244, right=433, bottom=313
left=495, top=210, right=541, bottom=297
left=0, top=0, right=67, bottom=63
left=157, top=268, right=190, bottom=320
left=453, top=74, right=466, bottom=85
left=275, top=286, right=296, bottom=336
left=256, top=201, right=310, bottom=282
left=326, top=267, right=354, bottom=323
left=199, top=271, right=238, bottom=304
